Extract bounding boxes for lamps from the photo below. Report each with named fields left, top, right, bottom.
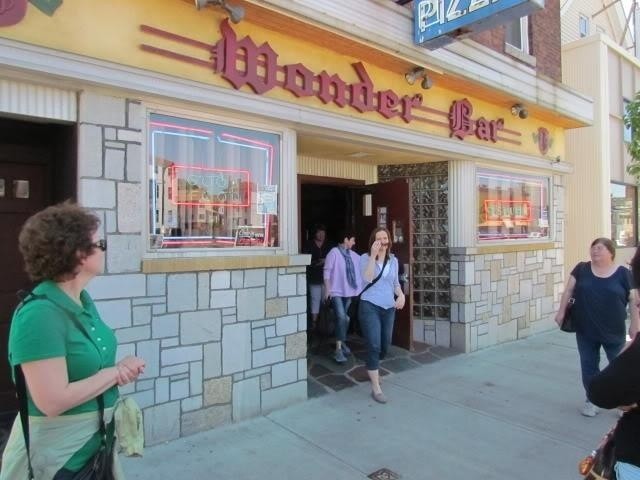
left=404, top=66, right=432, bottom=90
left=510, top=102, right=529, bottom=120
left=194, top=0, right=247, bottom=25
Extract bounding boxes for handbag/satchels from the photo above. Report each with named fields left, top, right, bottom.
left=560, top=295, right=579, bottom=333
left=73, top=446, right=107, bottom=480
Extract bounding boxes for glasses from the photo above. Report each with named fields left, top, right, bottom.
left=91, top=240, right=106, bottom=251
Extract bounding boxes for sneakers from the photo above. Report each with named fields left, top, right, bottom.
left=332, top=350, right=347, bottom=362
left=372, top=390, right=387, bottom=403
left=341, top=343, right=350, bottom=354
left=582, top=402, right=600, bottom=416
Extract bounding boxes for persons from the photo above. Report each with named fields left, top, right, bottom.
left=0, top=201, right=147, bottom=480
left=581, top=332, right=640, bottom=479
left=355, top=226, right=407, bottom=404
left=554, top=237, right=640, bottom=417
left=627, top=243, right=640, bottom=315
left=321, top=230, right=362, bottom=364
left=301, top=224, right=331, bottom=336
